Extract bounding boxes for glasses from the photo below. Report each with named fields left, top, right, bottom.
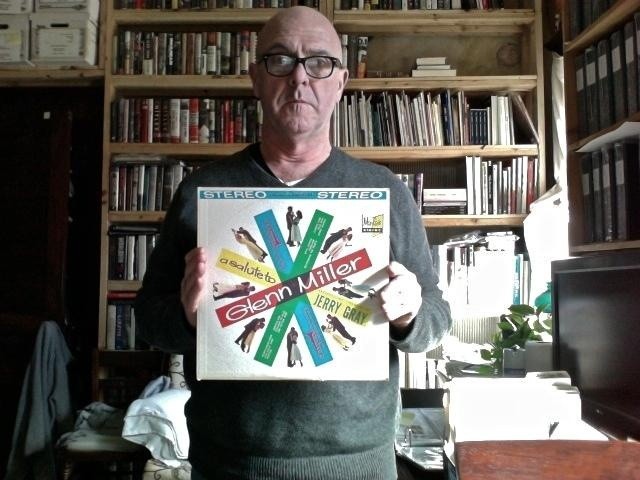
left=255, top=54, right=344, bottom=79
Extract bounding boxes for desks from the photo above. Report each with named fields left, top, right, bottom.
left=449, top=418, right=640, bottom=480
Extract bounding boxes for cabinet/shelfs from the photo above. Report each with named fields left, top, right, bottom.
left=95, top=0, right=640, bottom=404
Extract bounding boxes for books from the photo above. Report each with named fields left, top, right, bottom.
left=431, top=231, right=532, bottom=311
left=330, top=90, right=541, bottom=148
left=110, top=97, right=262, bottom=144
left=336, top=1, right=522, bottom=11
left=106, top=291, right=139, bottom=348
left=112, top=30, right=258, bottom=76
left=109, top=225, right=161, bottom=280
left=109, top=155, right=201, bottom=211
left=397, top=156, right=539, bottom=215
left=112, top=1, right=317, bottom=11
left=338, top=32, right=454, bottom=78
left=195, top=187, right=392, bottom=384
left=561, top=0, right=637, bottom=247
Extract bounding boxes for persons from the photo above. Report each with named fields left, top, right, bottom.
left=287, top=327, right=303, bottom=367
left=285, top=206, right=303, bottom=247
left=333, top=279, right=376, bottom=300
left=320, top=226, right=353, bottom=262
left=320, top=314, right=356, bottom=350
left=231, top=226, right=269, bottom=264
left=213, top=282, right=255, bottom=301
left=235, top=317, right=265, bottom=353
left=135, top=5, right=453, bottom=478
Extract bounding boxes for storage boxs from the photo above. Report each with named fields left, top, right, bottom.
left=0, top=0, right=96, bottom=66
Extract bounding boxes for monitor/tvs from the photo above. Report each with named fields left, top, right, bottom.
left=551, top=250, right=640, bottom=442
